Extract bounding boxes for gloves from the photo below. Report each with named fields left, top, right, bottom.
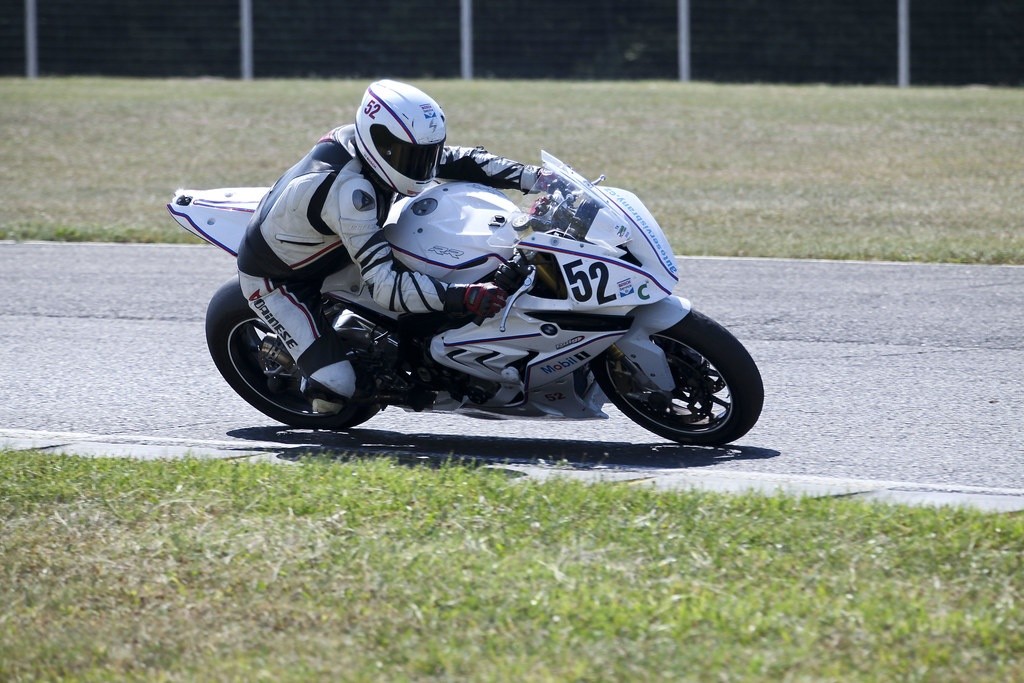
left=520, top=165, right=575, bottom=195
left=445, top=281, right=506, bottom=319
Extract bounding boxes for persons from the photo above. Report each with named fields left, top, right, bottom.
left=237, top=79, right=577, bottom=419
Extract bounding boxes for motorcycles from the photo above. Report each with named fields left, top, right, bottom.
left=167, top=148, right=765, bottom=446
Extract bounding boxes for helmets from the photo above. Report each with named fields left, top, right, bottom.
left=355, top=79, right=446, bottom=197
